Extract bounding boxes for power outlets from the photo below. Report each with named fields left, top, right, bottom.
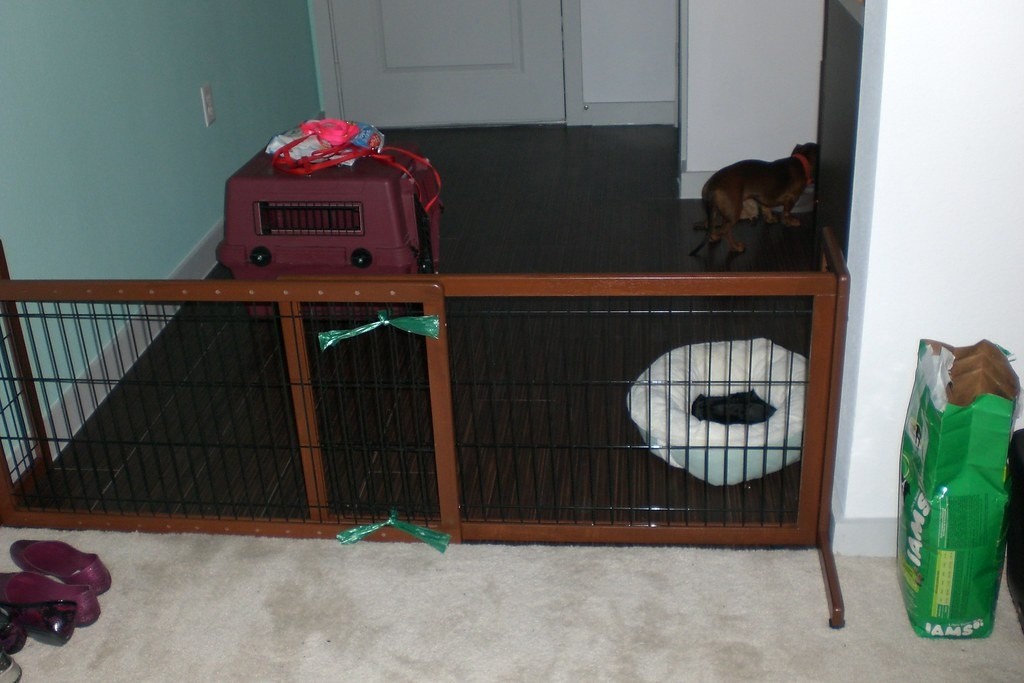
left=200, top=81, right=216, bottom=128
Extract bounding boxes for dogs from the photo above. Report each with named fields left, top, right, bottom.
left=689, top=139, right=822, bottom=257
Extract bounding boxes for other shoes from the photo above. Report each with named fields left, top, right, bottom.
left=0, top=540, right=112, bottom=627
left=0, top=651, right=23, bottom=682
left=0, top=600, right=78, bottom=655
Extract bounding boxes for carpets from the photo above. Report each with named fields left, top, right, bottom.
left=0, top=528, right=1024, bottom=683
